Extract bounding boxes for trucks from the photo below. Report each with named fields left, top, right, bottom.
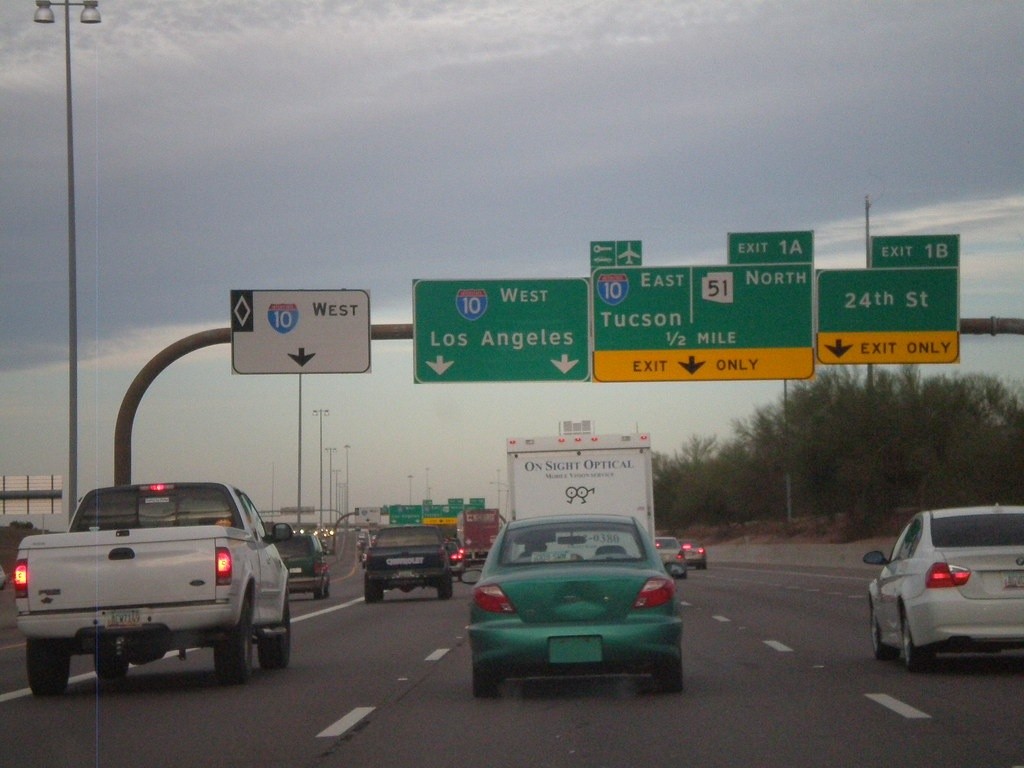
left=507, top=435, right=666, bottom=545
left=462, top=508, right=508, bottom=566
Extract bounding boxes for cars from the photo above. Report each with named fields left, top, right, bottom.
left=460, top=513, right=688, bottom=692
left=655, top=536, right=690, bottom=576
left=859, top=503, right=1024, bottom=670
left=441, top=536, right=467, bottom=574
left=682, top=539, right=709, bottom=564
left=270, top=529, right=341, bottom=595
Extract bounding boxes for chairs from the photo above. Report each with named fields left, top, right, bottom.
left=595, top=545, right=626, bottom=555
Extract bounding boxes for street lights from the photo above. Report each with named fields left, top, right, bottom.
left=324, top=447, right=337, bottom=529
left=312, top=409, right=331, bottom=530
left=496, top=467, right=503, bottom=508
left=32, top=0, right=103, bottom=537
left=344, top=444, right=353, bottom=525
left=407, top=475, right=417, bottom=506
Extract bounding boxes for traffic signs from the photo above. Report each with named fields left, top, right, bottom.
left=424, top=504, right=480, bottom=527
left=589, top=266, right=813, bottom=382
left=410, top=274, right=590, bottom=384
left=388, top=504, right=426, bottom=529
left=804, top=262, right=962, bottom=367
left=228, top=286, right=371, bottom=379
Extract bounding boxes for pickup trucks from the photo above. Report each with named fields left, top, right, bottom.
left=11, top=481, right=293, bottom=697
left=363, top=525, right=454, bottom=603
